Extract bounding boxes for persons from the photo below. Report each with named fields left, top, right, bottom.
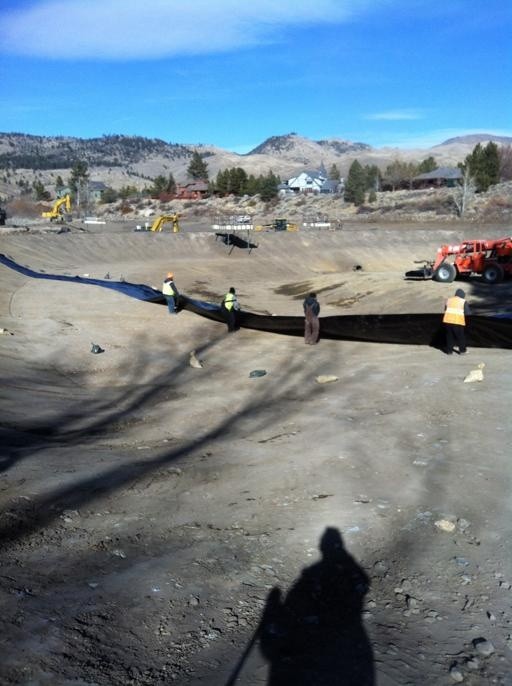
left=442, top=289, right=472, bottom=355
left=303, top=293, right=320, bottom=344
left=222, top=287, right=240, bottom=332
left=163, top=271, right=179, bottom=314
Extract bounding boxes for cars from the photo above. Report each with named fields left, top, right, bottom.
left=237, top=216, right=250, bottom=222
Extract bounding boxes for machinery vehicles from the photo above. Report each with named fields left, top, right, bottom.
left=134, top=212, right=180, bottom=232
left=255, top=218, right=298, bottom=231
left=406, top=238, right=512, bottom=284
left=42, top=194, right=72, bottom=222
